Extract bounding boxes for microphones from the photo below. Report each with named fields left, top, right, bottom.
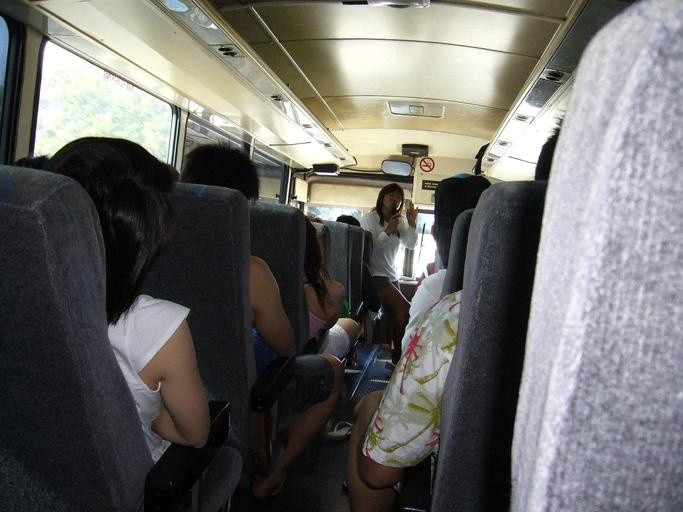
left=392, top=202, right=398, bottom=219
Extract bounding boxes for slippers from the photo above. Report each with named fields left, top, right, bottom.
left=326, top=420, right=352, bottom=440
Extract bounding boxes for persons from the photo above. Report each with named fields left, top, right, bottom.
left=44, top=135, right=210, bottom=467
left=340, top=114, right=567, bottom=510
left=301, top=216, right=346, bottom=438
left=178, top=138, right=345, bottom=500
left=400, top=173, right=491, bottom=356
left=336, top=215, right=380, bottom=344
left=356, top=182, right=419, bottom=366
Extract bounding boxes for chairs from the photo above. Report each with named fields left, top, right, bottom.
left=407, top=0, right=682, bottom=511
left=0, top=162, right=385, bottom=511
